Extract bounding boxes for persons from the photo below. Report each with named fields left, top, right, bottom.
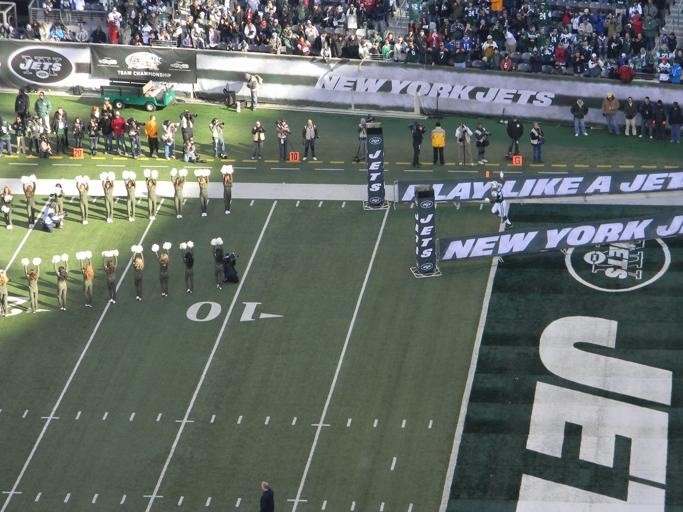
left=260, top=481, right=275, bottom=512
left=244, top=73, right=261, bottom=112
left=303, top=119, right=319, bottom=160
left=2, top=164, right=233, bottom=233
left=251, top=120, right=265, bottom=159
left=152, top=241, right=172, bottom=297
left=131, top=245, right=144, bottom=301
left=488, top=181, right=512, bottom=233
left=0, top=250, right=94, bottom=317
left=180, top=240, right=194, bottom=294
left=210, top=237, right=224, bottom=290
left=0, top=0, right=683, bottom=81
left=276, top=119, right=291, bottom=161
left=572, top=93, right=683, bottom=144
left=411, top=115, right=545, bottom=166
left=1, top=87, right=198, bottom=162
left=210, top=118, right=226, bottom=158
left=101, top=249, right=119, bottom=304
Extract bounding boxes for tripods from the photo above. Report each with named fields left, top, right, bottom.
left=354, top=125, right=367, bottom=162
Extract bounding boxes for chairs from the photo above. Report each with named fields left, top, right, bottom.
left=443, top=0, right=627, bottom=81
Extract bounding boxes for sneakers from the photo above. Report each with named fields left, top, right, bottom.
left=6, top=223, right=33, bottom=229
left=478, top=158, right=487, bottom=165
left=574, top=132, right=681, bottom=145
left=89, top=148, right=318, bottom=162
left=148, top=210, right=230, bottom=220
left=82, top=217, right=134, bottom=224
